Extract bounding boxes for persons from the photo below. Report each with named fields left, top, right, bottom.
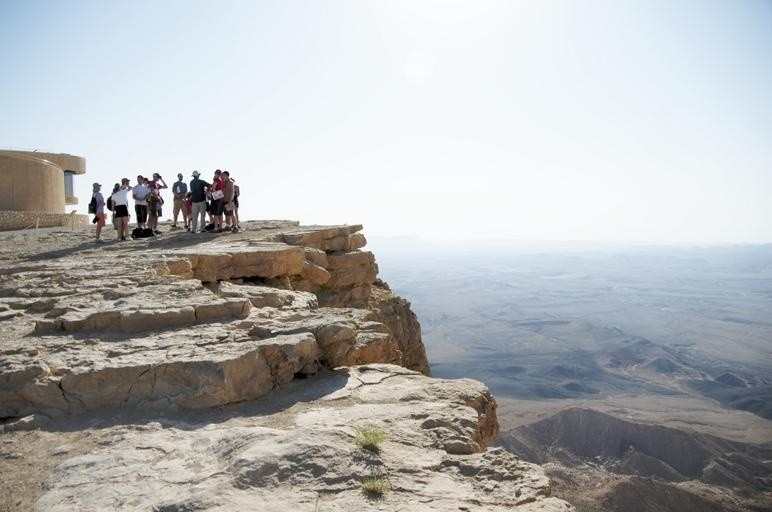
left=88, top=173, right=168, bottom=243
left=171, top=169, right=240, bottom=234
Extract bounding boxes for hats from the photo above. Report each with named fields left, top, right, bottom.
left=192, top=170, right=201, bottom=178
left=122, top=178, right=131, bottom=182
left=93, top=183, right=102, bottom=187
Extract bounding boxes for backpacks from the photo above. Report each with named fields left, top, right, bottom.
left=88, top=198, right=96, bottom=213
left=132, top=228, right=154, bottom=238
left=107, top=197, right=112, bottom=209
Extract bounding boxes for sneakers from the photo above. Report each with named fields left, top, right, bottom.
left=114, top=236, right=133, bottom=241
left=96, top=238, right=104, bottom=242
left=209, top=224, right=240, bottom=233
